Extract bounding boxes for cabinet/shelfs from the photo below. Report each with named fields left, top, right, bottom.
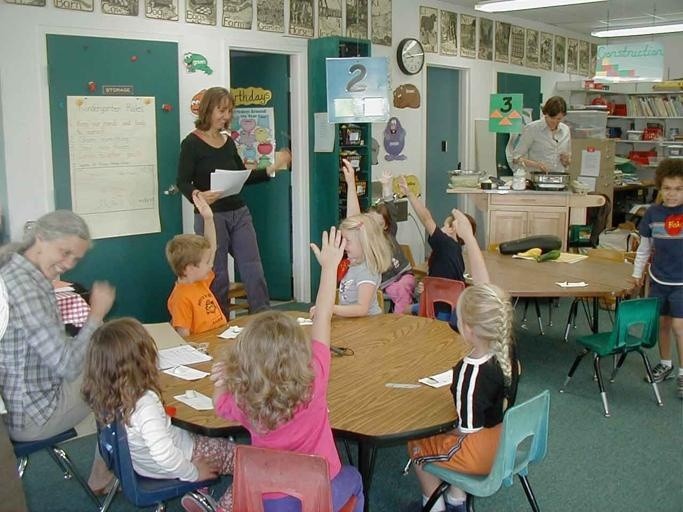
left=475, top=119, right=514, bottom=181
left=567, top=90, right=683, bottom=176
left=459, top=193, right=569, bottom=256
left=308, top=35, right=372, bottom=304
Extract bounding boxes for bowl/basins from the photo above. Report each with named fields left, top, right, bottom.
left=627, top=131, right=644, bottom=140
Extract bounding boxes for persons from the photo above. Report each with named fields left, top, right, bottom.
left=308, top=157, right=392, bottom=321
left=398, top=175, right=477, bottom=322
left=511, top=95, right=573, bottom=191
left=79, top=316, right=240, bottom=512
left=0, top=209, right=117, bottom=444
left=362, top=205, right=418, bottom=316
left=209, top=224, right=365, bottom=511
left=176, top=87, right=293, bottom=321
left=406, top=207, right=522, bottom=511
left=629, top=157, right=683, bottom=398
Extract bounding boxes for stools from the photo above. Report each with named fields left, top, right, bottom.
left=226, top=281, right=251, bottom=321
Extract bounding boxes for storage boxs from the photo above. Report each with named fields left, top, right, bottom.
left=566, top=110, right=608, bottom=138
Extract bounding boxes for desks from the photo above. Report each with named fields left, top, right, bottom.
left=156, top=310, right=474, bottom=512
left=614, top=181, right=654, bottom=229
left=413, top=254, right=637, bottom=343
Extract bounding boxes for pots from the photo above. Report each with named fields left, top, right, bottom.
left=447, top=170, right=481, bottom=188
left=530, top=172, right=569, bottom=190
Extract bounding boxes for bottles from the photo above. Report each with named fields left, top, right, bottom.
left=481, top=183, right=491, bottom=189
left=512, top=169, right=527, bottom=190
left=670, top=128, right=679, bottom=136
left=671, top=149, right=680, bottom=156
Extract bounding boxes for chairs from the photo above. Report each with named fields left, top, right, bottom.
left=335, top=289, right=384, bottom=315
left=420, top=389, right=551, bottom=511
left=559, top=297, right=663, bottom=418
left=569, top=193, right=611, bottom=253
left=0, top=404, right=222, bottom=511
left=626, top=189, right=663, bottom=228
left=231, top=446, right=357, bottom=511
left=513, top=249, right=625, bottom=343
left=400, top=244, right=428, bottom=278
left=419, top=277, right=465, bottom=323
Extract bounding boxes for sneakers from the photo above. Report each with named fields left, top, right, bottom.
left=645, top=363, right=673, bottom=384
left=180, top=490, right=216, bottom=512
left=405, top=497, right=467, bottom=512
left=676, top=375, right=683, bottom=398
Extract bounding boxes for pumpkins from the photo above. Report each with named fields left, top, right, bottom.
left=497, top=235, right=562, bottom=253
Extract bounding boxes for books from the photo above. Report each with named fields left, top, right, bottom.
left=140, top=322, right=214, bottom=370
left=626, top=93, right=683, bottom=118
left=575, top=128, right=592, bottom=138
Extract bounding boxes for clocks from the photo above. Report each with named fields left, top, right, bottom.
left=397, top=38, right=424, bottom=75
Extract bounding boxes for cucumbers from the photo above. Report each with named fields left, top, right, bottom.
left=537, top=249, right=560, bottom=263
